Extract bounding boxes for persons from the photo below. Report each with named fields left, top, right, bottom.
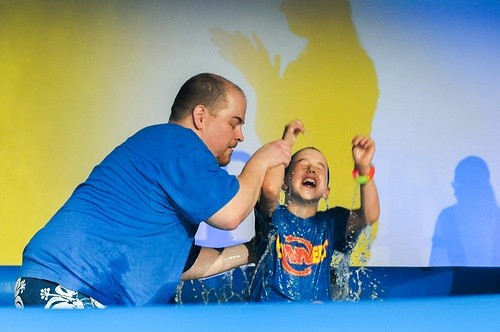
left=249, top=118, right=382, bottom=303
left=14, top=72, right=293, bottom=312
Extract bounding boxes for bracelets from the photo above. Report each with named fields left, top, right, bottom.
left=352, top=163, right=376, bottom=184
